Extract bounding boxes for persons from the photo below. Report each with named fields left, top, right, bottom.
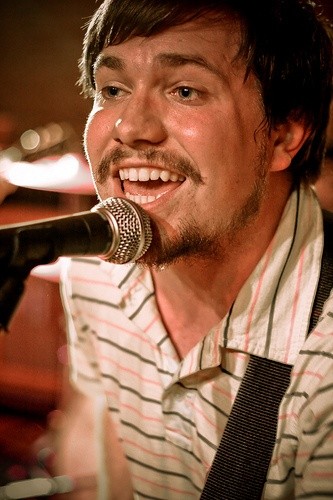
left=18, top=0, right=333, bottom=499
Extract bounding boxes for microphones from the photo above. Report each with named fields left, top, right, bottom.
left=1, top=196, right=152, bottom=269
